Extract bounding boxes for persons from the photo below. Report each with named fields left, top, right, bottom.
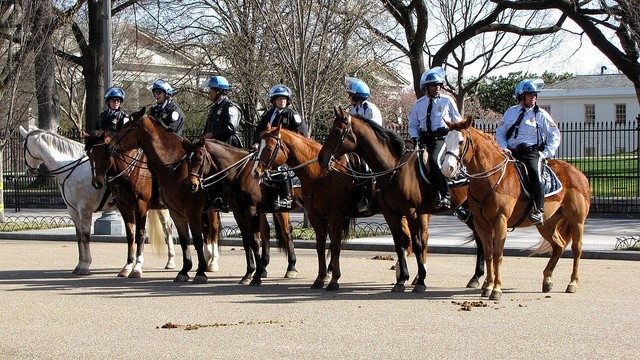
left=80, top=87, right=129, bottom=142
left=251, top=85, right=308, bottom=209
left=147, top=79, right=186, bottom=208
left=407, top=66, right=462, bottom=210
left=342, top=78, right=383, bottom=216
left=200, top=74, right=242, bottom=213
left=496, top=79, right=560, bottom=227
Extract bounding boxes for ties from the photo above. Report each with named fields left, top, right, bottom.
left=426, top=99, right=433, bottom=135
left=157, top=106, right=162, bottom=118
left=272, top=111, right=279, bottom=127
left=356, top=106, right=359, bottom=114
left=506, top=108, right=526, bottom=140
left=108, top=111, right=112, bottom=116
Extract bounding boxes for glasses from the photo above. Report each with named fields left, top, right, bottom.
left=349, top=94, right=352, bottom=96
left=430, top=84, right=442, bottom=86
left=110, top=98, right=121, bottom=102
left=153, top=91, right=162, bottom=95
left=527, top=93, right=536, bottom=96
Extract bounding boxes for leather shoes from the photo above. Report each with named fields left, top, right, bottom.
left=274, top=196, right=293, bottom=205
left=108, top=197, right=117, bottom=207
left=454, top=207, right=474, bottom=229
left=437, top=197, right=452, bottom=208
left=359, top=196, right=373, bottom=208
left=214, top=195, right=229, bottom=213
left=529, top=211, right=544, bottom=226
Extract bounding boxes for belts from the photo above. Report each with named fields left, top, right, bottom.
left=513, top=145, right=538, bottom=152
left=425, top=132, right=437, bottom=136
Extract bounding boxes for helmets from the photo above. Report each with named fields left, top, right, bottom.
left=207, top=75, right=230, bottom=90
left=152, top=79, right=173, bottom=96
left=104, top=87, right=126, bottom=103
left=515, top=78, right=544, bottom=94
left=269, top=84, right=292, bottom=98
left=344, top=77, right=370, bottom=98
left=420, top=66, right=446, bottom=89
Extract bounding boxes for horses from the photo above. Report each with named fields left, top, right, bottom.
left=439, top=116, right=592, bottom=300
left=80, top=128, right=222, bottom=279
left=250, top=121, right=429, bottom=290
left=318, top=104, right=486, bottom=292
left=104, top=106, right=289, bottom=286
left=19, top=123, right=179, bottom=275
left=182, top=135, right=359, bottom=288
